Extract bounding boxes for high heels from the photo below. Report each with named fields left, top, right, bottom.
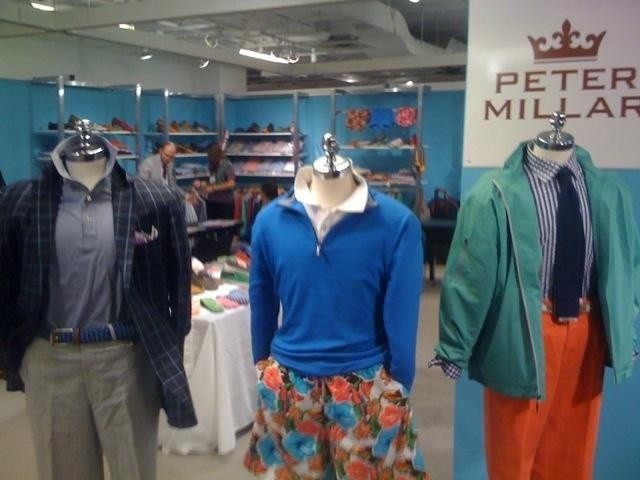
left=112, top=117, right=133, bottom=132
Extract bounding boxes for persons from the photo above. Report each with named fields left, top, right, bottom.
left=244, top=134, right=433, bottom=480
left=1, top=120, right=199, bottom=480
left=194, top=143, right=236, bottom=263
left=240, top=179, right=285, bottom=257
left=428, top=114, right=640, bottom=479
left=139, top=140, right=198, bottom=207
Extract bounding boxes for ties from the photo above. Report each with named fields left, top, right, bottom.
left=552, top=167, right=584, bottom=317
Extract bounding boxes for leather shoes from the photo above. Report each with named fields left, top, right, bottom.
left=156, top=119, right=209, bottom=133
left=176, top=142, right=204, bottom=153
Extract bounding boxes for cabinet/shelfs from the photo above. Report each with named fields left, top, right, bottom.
left=31, top=74, right=307, bottom=181
left=330, top=84, right=425, bottom=228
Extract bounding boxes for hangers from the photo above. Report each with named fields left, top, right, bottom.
left=233, top=182, right=284, bottom=200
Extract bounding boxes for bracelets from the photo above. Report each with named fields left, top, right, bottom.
left=211, top=184, right=215, bottom=192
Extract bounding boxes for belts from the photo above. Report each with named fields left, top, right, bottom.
left=34, top=322, right=136, bottom=347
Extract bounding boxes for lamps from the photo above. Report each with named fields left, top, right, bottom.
left=204, top=24, right=223, bottom=48
left=118, top=23, right=136, bottom=32
left=139, top=47, right=152, bottom=61
left=310, top=46, right=318, bottom=62
left=239, top=31, right=300, bottom=66
left=30, top=0, right=55, bottom=13
left=198, top=58, right=209, bottom=69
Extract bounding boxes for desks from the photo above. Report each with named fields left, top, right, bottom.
left=156, top=257, right=285, bottom=459
left=421, top=217, right=457, bottom=280
